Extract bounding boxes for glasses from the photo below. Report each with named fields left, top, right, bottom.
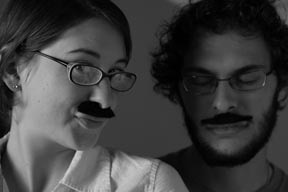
left=31, top=51, right=137, bottom=92
left=170, top=63, right=273, bottom=95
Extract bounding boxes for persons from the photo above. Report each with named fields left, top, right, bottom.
left=0, top=0, right=189, bottom=192
left=150, top=0, right=288, bottom=192
